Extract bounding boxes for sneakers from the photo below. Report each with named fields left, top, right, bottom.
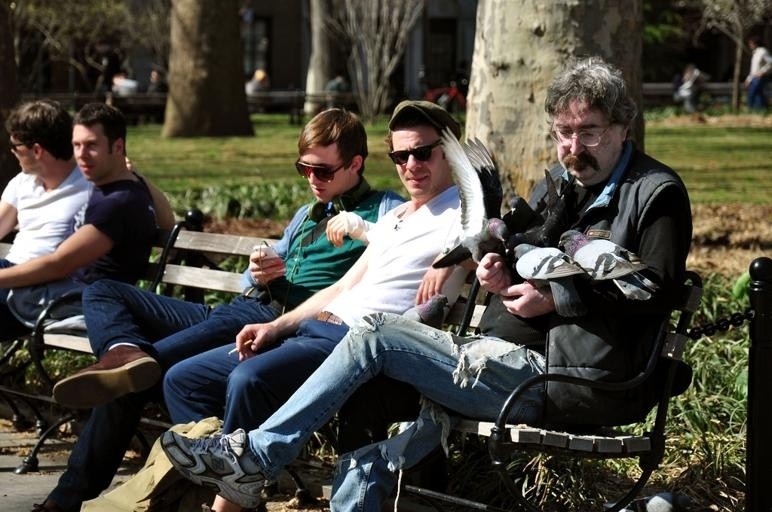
left=161, top=429, right=270, bottom=509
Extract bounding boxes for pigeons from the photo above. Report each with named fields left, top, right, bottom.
left=432, top=126, right=508, bottom=269
left=503, top=196, right=544, bottom=237
left=513, top=243, right=588, bottom=317
left=402, top=293, right=451, bottom=330
left=559, top=229, right=659, bottom=301
left=603, top=492, right=693, bottom=512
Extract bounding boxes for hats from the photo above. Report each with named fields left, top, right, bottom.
left=389, top=100, right=461, bottom=139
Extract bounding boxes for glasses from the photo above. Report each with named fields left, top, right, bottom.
left=552, top=119, right=614, bottom=148
left=294, top=156, right=348, bottom=182
left=8, top=140, right=24, bottom=148
left=388, top=141, right=443, bottom=166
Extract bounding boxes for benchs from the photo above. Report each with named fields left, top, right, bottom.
left=642, top=81, right=746, bottom=122
left=72, top=89, right=306, bottom=126
left=0, top=212, right=704, bottom=512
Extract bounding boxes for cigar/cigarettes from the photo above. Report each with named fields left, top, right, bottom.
left=228, top=339, right=253, bottom=355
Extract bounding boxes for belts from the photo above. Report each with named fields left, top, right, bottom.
left=318, top=312, right=345, bottom=326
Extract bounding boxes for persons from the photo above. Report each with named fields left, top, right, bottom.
left=163, top=100, right=480, bottom=512
left=111, top=69, right=168, bottom=97
left=321, top=76, right=342, bottom=105
left=0, top=101, right=158, bottom=343
left=743, top=36, right=772, bottom=113
left=32, top=107, right=406, bottom=512
left=0, top=99, right=176, bottom=265
left=160, top=55, right=692, bottom=512
left=674, top=60, right=706, bottom=107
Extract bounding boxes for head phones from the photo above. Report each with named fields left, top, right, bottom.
left=308, top=177, right=371, bottom=223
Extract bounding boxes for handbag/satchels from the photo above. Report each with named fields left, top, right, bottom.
left=81, top=416, right=225, bottom=512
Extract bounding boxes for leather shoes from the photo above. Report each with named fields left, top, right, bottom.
left=54, top=345, right=161, bottom=409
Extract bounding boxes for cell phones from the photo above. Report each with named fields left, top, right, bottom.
left=253, top=245, right=288, bottom=274
